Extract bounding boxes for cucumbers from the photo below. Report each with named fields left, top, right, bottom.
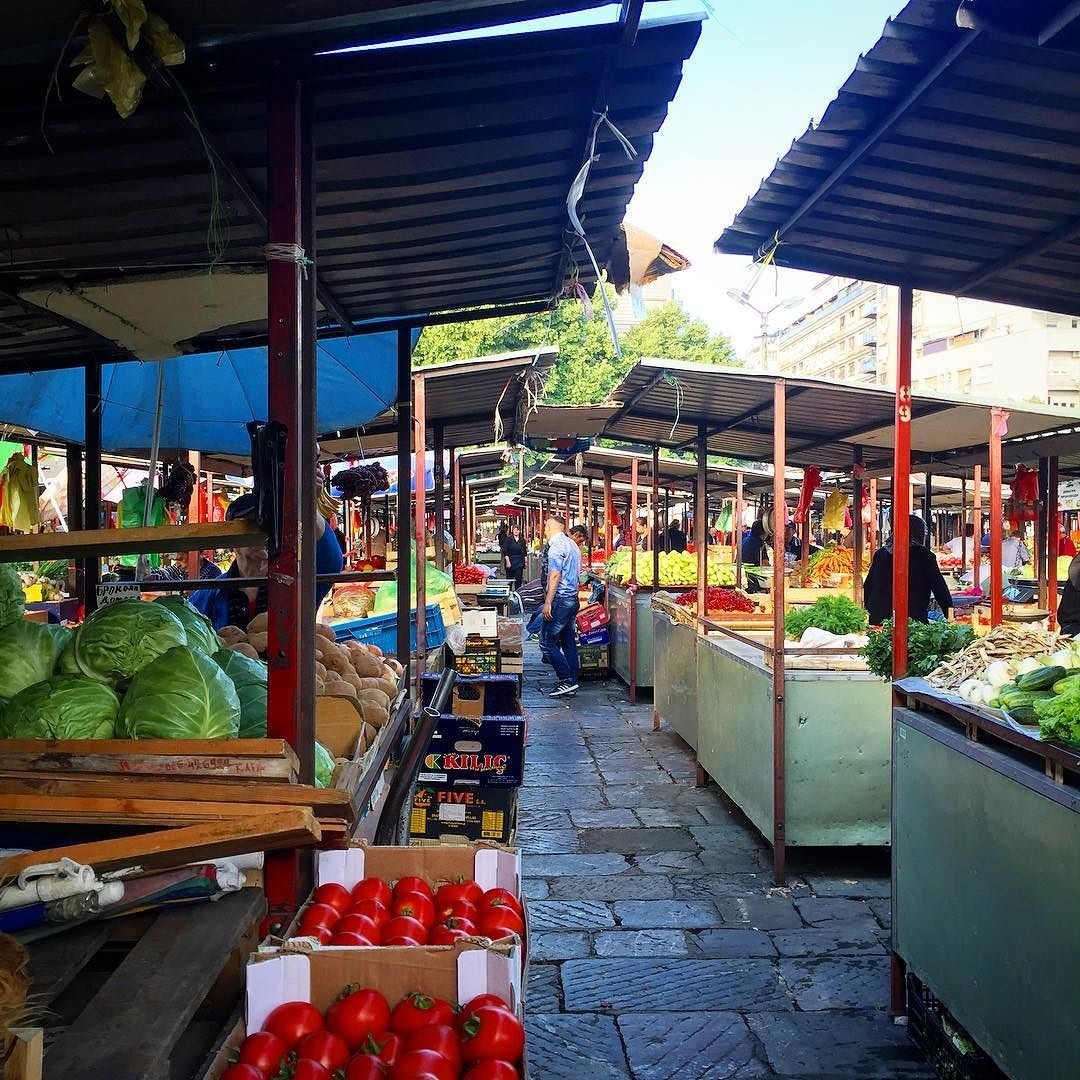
left=989, top=660, right=1079, bottom=726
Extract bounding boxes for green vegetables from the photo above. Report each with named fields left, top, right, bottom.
left=856, top=616, right=981, bottom=682
left=784, top=594, right=870, bottom=642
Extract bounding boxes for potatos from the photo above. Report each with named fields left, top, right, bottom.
left=218, top=611, right=402, bottom=745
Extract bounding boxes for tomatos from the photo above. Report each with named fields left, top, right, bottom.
left=455, top=564, right=483, bottom=584
left=939, top=558, right=962, bottom=566
left=216, top=983, right=525, bottom=1079
left=292, top=875, right=525, bottom=946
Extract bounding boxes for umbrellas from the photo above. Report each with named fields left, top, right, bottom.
left=0, top=314, right=433, bottom=588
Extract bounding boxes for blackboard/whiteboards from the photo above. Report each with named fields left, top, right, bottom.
left=95, top=585, right=141, bottom=606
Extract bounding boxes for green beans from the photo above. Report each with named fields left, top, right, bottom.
left=925, top=625, right=1077, bottom=691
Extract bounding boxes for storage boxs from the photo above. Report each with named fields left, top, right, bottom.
left=329, top=604, right=446, bottom=656
left=407, top=672, right=526, bottom=844
left=248, top=848, right=523, bottom=1009
left=574, top=603, right=611, bottom=676
left=454, top=606, right=524, bottom=671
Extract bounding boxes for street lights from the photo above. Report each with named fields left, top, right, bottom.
left=726, top=287, right=806, bottom=368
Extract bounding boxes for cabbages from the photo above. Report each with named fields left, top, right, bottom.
left=0, top=565, right=332, bottom=789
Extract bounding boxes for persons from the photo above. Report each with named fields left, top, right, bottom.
left=540, top=525, right=588, bottom=663
left=188, top=467, right=344, bottom=630
left=943, top=524, right=974, bottom=566
left=111, top=546, right=222, bottom=598
left=538, top=515, right=582, bottom=697
left=1057, top=550, right=1080, bottom=638
left=1001, top=530, right=1028, bottom=569
left=503, top=524, right=528, bottom=591
left=498, top=523, right=510, bottom=576
left=863, top=515, right=953, bottom=626
left=1057, top=523, right=1077, bottom=558
left=616, top=516, right=802, bottom=566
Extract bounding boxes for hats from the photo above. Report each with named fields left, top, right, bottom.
left=785, top=520, right=797, bottom=532
left=225, top=493, right=260, bottom=522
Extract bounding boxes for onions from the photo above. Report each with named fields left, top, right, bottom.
left=959, top=650, right=1072, bottom=704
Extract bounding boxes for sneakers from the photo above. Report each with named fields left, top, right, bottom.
left=549, top=679, right=580, bottom=696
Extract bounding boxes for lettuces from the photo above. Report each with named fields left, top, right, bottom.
left=1033, top=677, right=1079, bottom=747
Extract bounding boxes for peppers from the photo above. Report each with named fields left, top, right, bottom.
left=672, top=586, right=756, bottom=614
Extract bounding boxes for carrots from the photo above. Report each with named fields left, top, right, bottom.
left=810, top=550, right=871, bottom=578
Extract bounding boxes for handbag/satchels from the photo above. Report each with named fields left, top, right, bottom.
left=1006, top=464, right=1040, bottom=522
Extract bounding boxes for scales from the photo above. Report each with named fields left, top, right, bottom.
left=1003, top=586, right=1064, bottom=604
left=476, top=577, right=516, bottom=596
left=953, top=597, right=982, bottom=616
